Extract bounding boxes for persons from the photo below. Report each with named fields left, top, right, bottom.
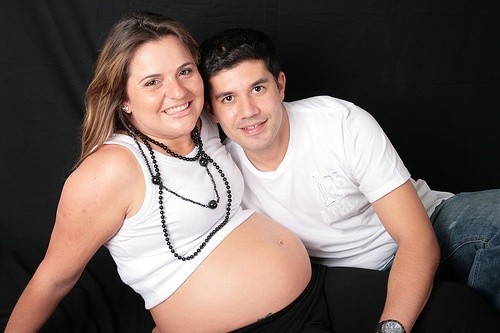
left=0, top=12, right=499, bottom=333
left=199, top=26, right=500, bottom=333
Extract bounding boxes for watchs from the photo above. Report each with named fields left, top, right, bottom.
left=373, top=319, right=407, bottom=333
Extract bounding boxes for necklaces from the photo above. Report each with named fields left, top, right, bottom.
left=126, top=122, right=233, bottom=261
